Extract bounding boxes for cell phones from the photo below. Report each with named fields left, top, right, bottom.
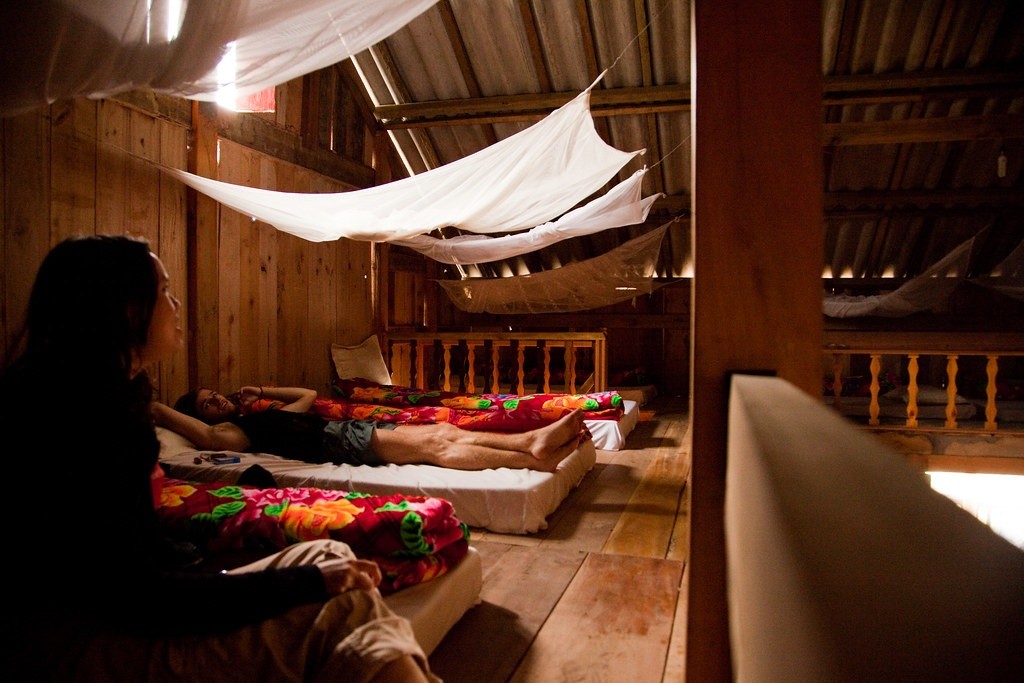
left=228, top=393, right=242, bottom=406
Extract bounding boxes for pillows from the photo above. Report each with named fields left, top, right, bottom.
left=430, top=374, right=485, bottom=387
left=882, top=383, right=969, bottom=406
left=156, top=425, right=196, bottom=459
left=330, top=333, right=393, bottom=386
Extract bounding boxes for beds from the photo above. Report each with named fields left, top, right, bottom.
left=155, top=384, right=657, bottom=660
left=822, top=395, right=1024, bottom=422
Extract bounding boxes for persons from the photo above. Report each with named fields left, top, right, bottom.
left=0, top=237, right=446, bottom=683
left=149, top=385, right=586, bottom=473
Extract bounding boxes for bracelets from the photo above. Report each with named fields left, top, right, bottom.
left=259, top=385, right=263, bottom=396
